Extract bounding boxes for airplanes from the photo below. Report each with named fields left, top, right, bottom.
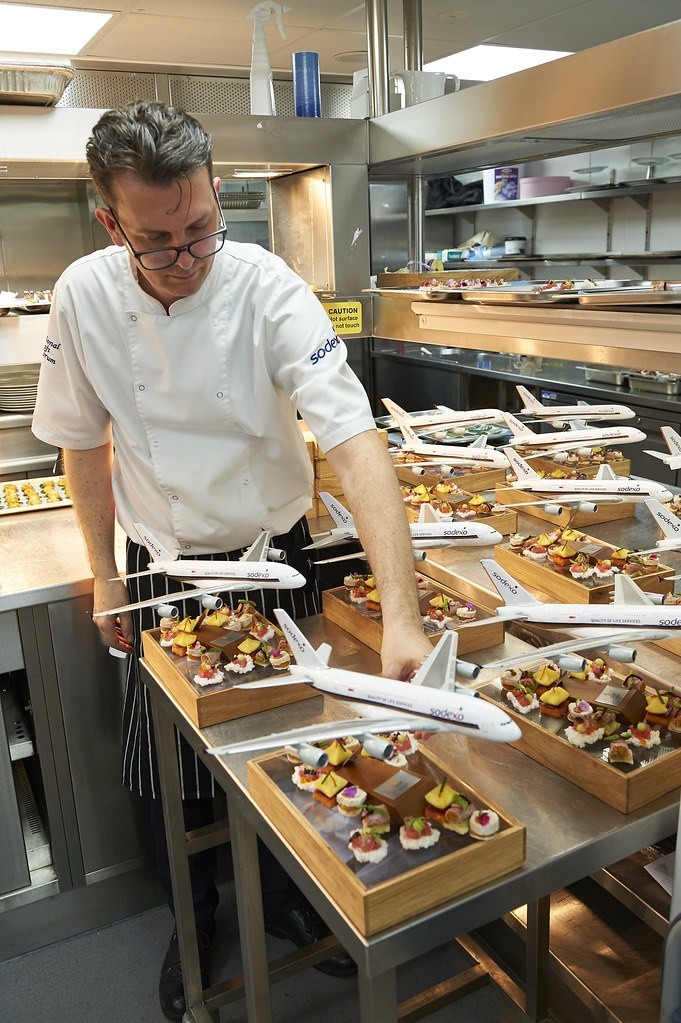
left=89, top=523, right=306, bottom=619
left=457, top=559, right=681, bottom=669
left=205, top=608, right=524, bottom=757
left=627, top=499, right=681, bottom=556
left=380, top=385, right=680, bottom=515
left=300, top=492, right=502, bottom=564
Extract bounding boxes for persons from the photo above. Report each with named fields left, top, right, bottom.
left=29, top=100, right=435, bottom=1022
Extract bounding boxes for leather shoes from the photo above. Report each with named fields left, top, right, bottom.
left=159, top=912, right=221, bottom=1021
left=261, top=876, right=358, bottom=979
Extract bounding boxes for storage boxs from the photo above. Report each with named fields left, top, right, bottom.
left=0, top=62, right=76, bottom=107
left=142, top=408, right=681, bottom=937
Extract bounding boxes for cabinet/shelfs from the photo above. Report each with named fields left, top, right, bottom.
left=374, top=357, right=681, bottom=490
left=0, top=18, right=681, bottom=367
left=1, top=589, right=155, bottom=918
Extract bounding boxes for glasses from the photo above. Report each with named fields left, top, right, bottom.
left=107, top=184, right=228, bottom=271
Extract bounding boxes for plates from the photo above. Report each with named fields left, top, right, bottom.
left=0, top=375, right=39, bottom=412
left=562, top=151, right=681, bottom=193
left=0, top=61, right=77, bottom=105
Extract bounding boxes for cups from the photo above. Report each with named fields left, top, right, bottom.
left=294, top=52, right=323, bottom=118
left=505, top=236, right=527, bottom=254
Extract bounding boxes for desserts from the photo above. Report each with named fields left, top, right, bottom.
left=539, top=277, right=602, bottom=292
left=418, top=278, right=506, bottom=291
left=159, top=441, right=681, bottom=867
left=2, top=478, right=71, bottom=509
left=653, top=281, right=673, bottom=291
